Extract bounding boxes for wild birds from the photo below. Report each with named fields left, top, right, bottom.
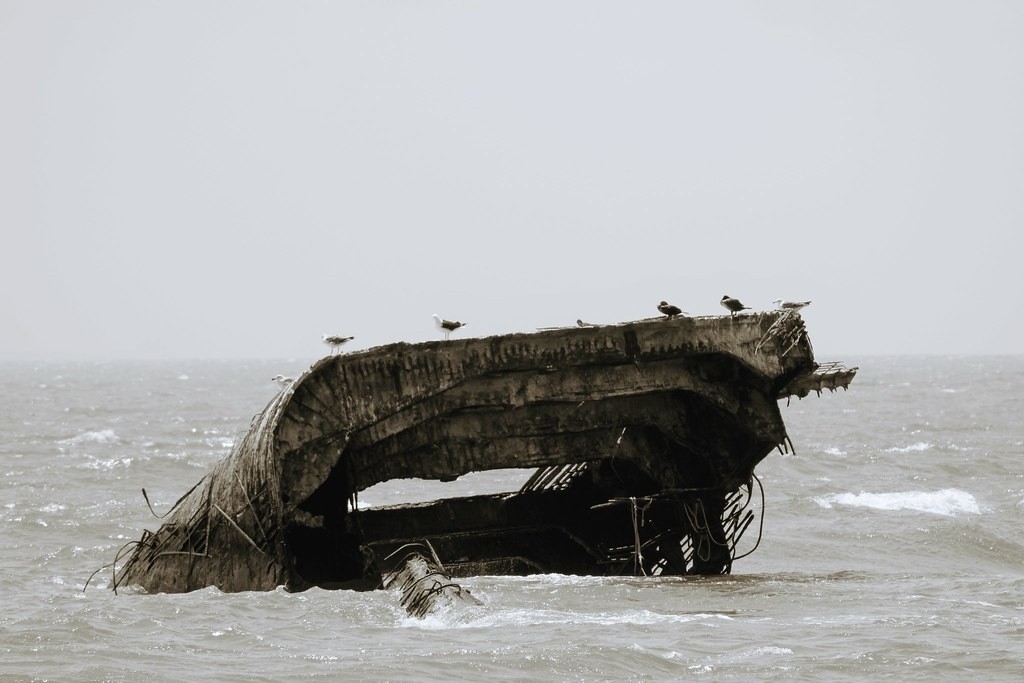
left=657, top=300, right=689, bottom=321
left=320, top=334, right=354, bottom=357
left=430, top=313, right=468, bottom=340
left=720, top=294, right=752, bottom=316
left=271, top=374, right=295, bottom=392
left=773, top=298, right=813, bottom=313
